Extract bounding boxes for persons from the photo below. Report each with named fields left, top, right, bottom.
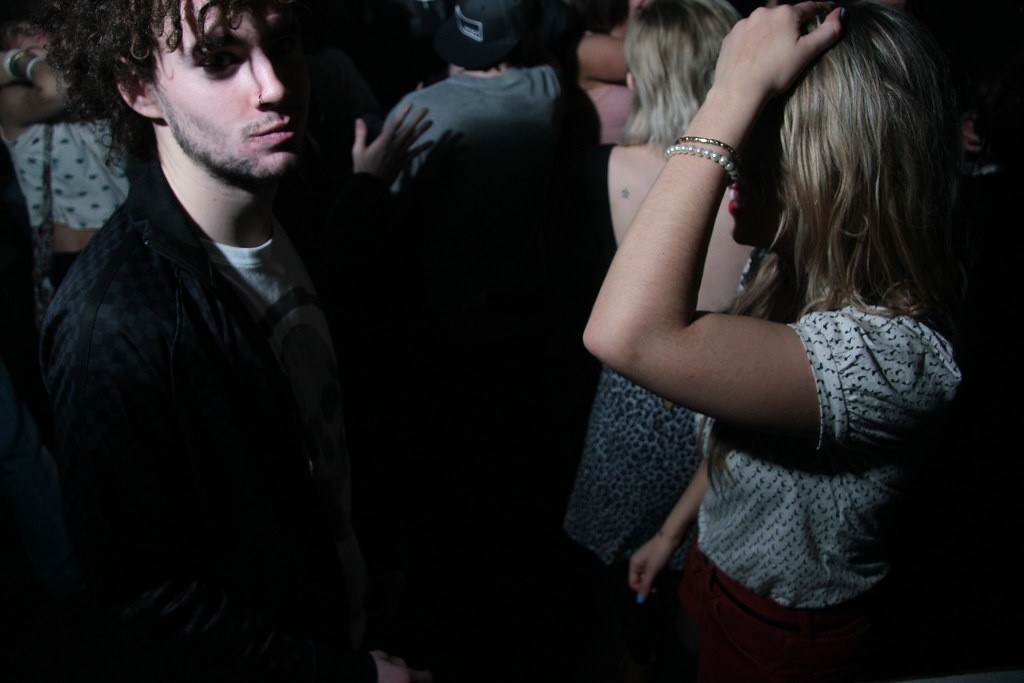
left=583, top=0, right=968, bottom=683
left=0, top=0, right=754, bottom=683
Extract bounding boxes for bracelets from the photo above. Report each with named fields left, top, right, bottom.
left=665, top=145, right=738, bottom=185
left=13, top=52, right=27, bottom=76
left=26, top=56, right=42, bottom=82
left=674, top=137, right=741, bottom=168
left=2, top=47, right=23, bottom=81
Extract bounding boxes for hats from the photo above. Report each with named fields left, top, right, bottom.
left=434, top=1, right=522, bottom=71
